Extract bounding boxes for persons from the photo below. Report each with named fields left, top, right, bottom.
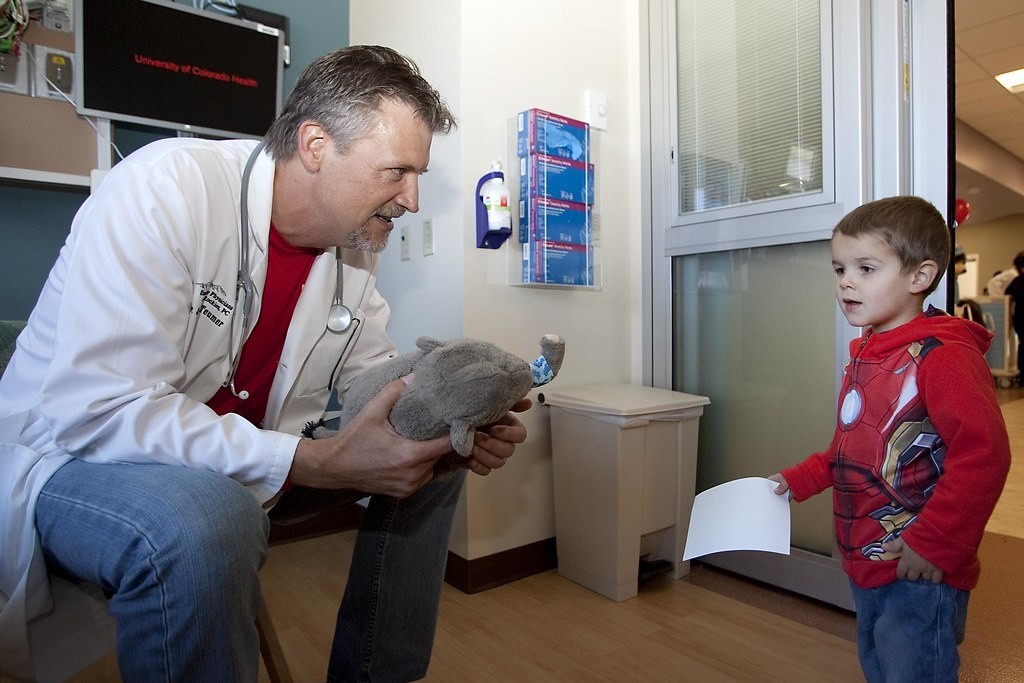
left=1004, top=250, right=1024, bottom=386
left=763, top=197, right=1012, bottom=683
left=0, top=45, right=533, bottom=682
left=987, top=266, right=1020, bottom=296
left=954, top=245, right=966, bottom=306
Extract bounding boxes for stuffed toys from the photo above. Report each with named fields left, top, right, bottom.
left=302, top=334, right=565, bottom=458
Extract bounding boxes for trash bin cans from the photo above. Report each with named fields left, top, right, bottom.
left=543, top=385, right=711, bottom=601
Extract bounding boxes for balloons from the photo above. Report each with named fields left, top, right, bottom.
left=955, top=197, right=970, bottom=225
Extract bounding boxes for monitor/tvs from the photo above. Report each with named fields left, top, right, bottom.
left=784, top=142, right=818, bottom=185
left=74, top=0, right=286, bottom=140
